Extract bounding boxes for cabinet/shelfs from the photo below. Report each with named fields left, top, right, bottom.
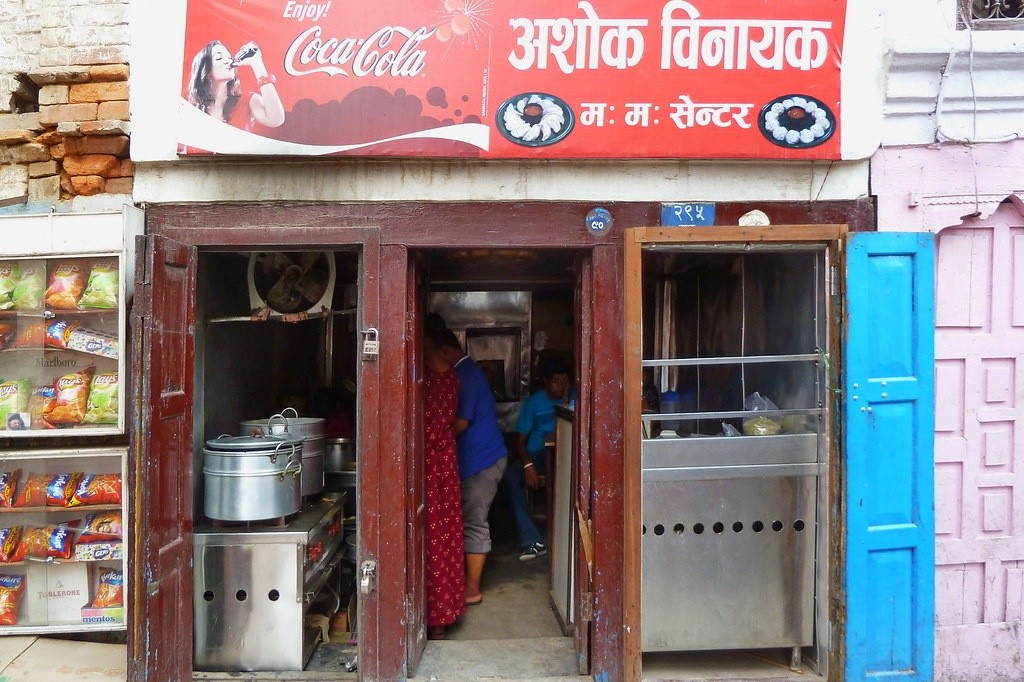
left=1, top=252, right=130, bottom=438
left=0, top=446, right=130, bottom=636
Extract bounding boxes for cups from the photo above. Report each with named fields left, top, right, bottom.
left=660, top=391, right=681, bottom=431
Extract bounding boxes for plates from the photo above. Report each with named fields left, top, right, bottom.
left=495, top=93, right=574, bottom=147
left=758, top=94, right=836, bottom=148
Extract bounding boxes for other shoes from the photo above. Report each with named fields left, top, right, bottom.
left=465, top=591, right=482, bottom=605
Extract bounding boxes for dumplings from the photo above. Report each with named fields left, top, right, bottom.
left=504, top=96, right=564, bottom=143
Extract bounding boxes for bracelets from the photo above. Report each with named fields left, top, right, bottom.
left=258, top=73, right=276, bottom=88
left=524, top=462, right=533, bottom=469
left=507, top=359, right=576, bottom=561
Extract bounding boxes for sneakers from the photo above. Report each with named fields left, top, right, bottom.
left=519, top=542, right=546, bottom=561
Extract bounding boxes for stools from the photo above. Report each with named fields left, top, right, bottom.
left=524, top=474, right=549, bottom=525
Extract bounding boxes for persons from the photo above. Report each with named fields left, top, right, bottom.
left=439, top=329, right=507, bottom=605
left=413, top=313, right=466, bottom=640
left=178, top=40, right=284, bottom=155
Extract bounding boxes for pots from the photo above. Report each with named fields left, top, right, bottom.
left=240, top=408, right=325, bottom=495
left=326, top=437, right=352, bottom=472
left=203, top=428, right=301, bottom=521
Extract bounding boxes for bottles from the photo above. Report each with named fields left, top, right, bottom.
left=231, top=40, right=259, bottom=67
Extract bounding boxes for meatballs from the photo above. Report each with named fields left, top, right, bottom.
left=765, top=97, right=828, bottom=144
